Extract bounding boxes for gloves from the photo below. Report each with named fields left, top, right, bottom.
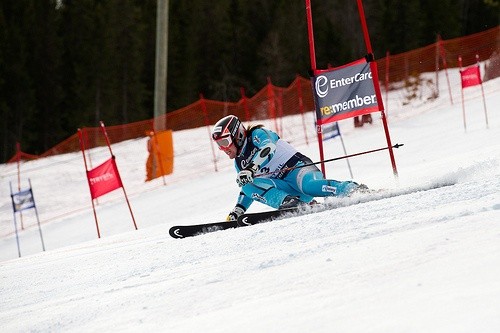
left=227, top=207, right=244, bottom=220
left=236, top=169, right=254, bottom=187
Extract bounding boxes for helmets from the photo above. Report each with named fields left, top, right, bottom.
left=212, top=114, right=246, bottom=147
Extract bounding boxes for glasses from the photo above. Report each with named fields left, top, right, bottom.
left=215, top=136, right=233, bottom=148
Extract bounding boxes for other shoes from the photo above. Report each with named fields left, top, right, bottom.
left=280, top=197, right=303, bottom=208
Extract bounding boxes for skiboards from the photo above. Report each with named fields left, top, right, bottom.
left=167, top=182, right=452, bottom=239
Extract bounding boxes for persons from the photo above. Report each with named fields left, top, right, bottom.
left=212, top=114, right=371, bottom=222
